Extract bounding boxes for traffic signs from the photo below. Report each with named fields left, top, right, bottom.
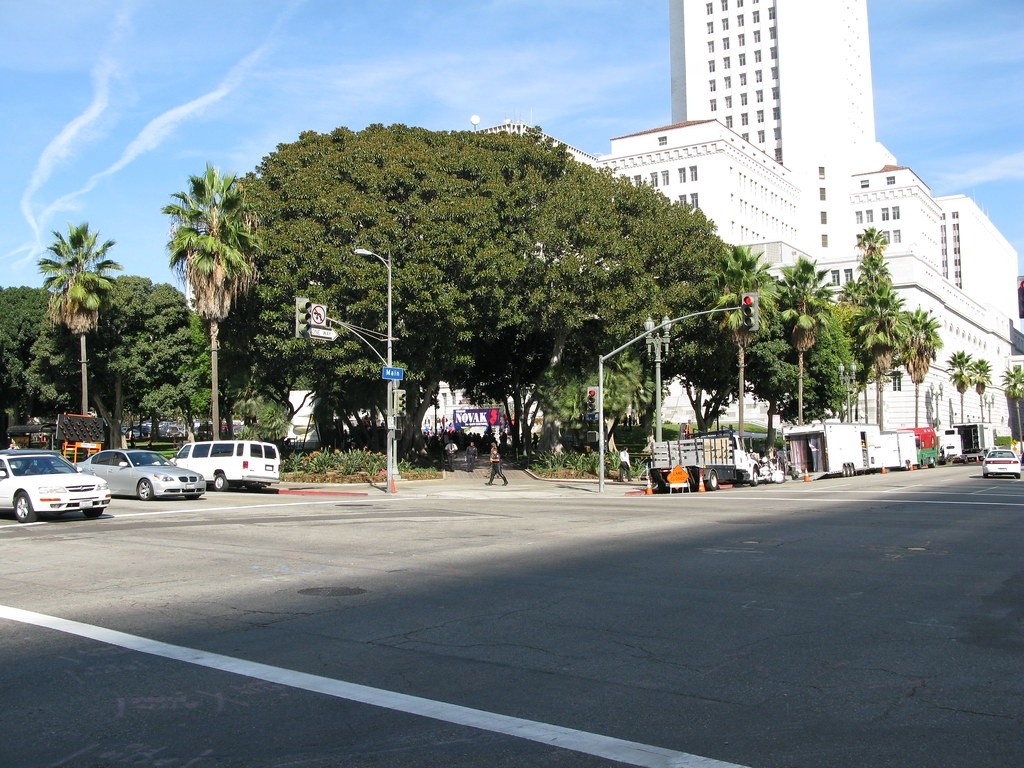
left=309, top=324, right=339, bottom=342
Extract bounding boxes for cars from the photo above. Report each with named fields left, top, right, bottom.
left=71, top=446, right=207, bottom=501
left=0, top=450, right=112, bottom=523
left=120, top=418, right=246, bottom=443
left=980, top=449, right=1022, bottom=479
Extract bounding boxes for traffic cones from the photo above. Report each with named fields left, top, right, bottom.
left=391, top=478, right=398, bottom=493
left=975, top=455, right=980, bottom=463
left=646, top=476, right=654, bottom=495
left=803, top=467, right=812, bottom=482
left=697, top=472, right=706, bottom=493
left=880, top=462, right=888, bottom=474
left=908, top=462, right=914, bottom=471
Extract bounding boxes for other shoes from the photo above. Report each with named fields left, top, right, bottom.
left=628, top=479, right=633, bottom=482
left=503, top=481, right=508, bottom=486
left=620, top=479, right=624, bottom=481
left=485, top=482, right=492, bottom=486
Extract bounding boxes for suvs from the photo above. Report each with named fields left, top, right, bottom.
left=165, top=440, right=281, bottom=492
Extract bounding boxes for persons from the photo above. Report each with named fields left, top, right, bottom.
left=486, top=442, right=501, bottom=479
left=8, top=443, right=14, bottom=450
left=501, top=432, right=507, bottom=444
left=442, top=430, right=497, bottom=450
left=619, top=447, right=634, bottom=482
left=464, top=441, right=478, bottom=472
left=623, top=413, right=632, bottom=431
left=532, top=433, right=538, bottom=449
left=485, top=449, right=509, bottom=486
left=444, top=439, right=458, bottom=472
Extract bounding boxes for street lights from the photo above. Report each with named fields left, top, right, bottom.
left=353, top=247, right=394, bottom=494
left=432, top=390, right=439, bottom=436
left=928, top=382, right=945, bottom=432
left=983, top=392, right=996, bottom=423
left=643, top=312, right=673, bottom=443
left=837, top=362, right=857, bottom=423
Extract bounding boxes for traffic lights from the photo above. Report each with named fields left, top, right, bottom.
left=295, top=297, right=312, bottom=339
left=586, top=385, right=600, bottom=413
left=587, top=430, right=598, bottom=442
left=392, top=389, right=407, bottom=417
left=741, top=292, right=759, bottom=331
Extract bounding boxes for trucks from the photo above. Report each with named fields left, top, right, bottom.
left=649, top=424, right=760, bottom=493
left=941, top=423, right=998, bottom=464
left=898, top=426, right=938, bottom=469
